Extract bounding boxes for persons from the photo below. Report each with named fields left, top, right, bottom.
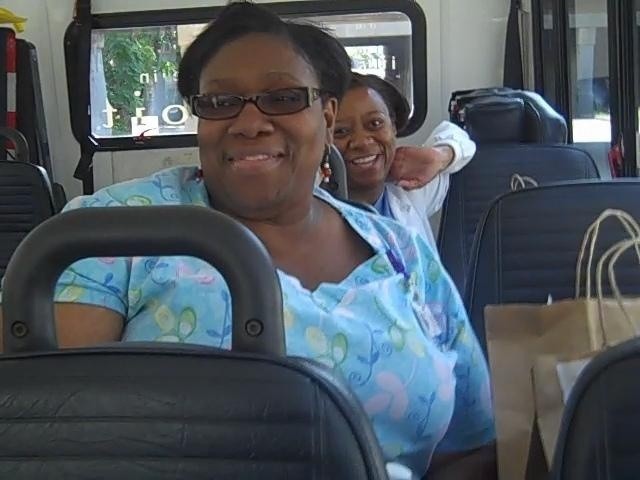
left=49, top=0, right=499, bottom=479
left=332, top=71, right=478, bottom=263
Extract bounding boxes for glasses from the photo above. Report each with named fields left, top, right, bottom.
left=191, top=86, right=325, bottom=120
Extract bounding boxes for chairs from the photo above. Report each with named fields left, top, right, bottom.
left=0, top=125, right=67, bottom=276
left=0, top=208, right=381, bottom=479
left=434, top=144, right=602, bottom=293
left=319, top=142, right=381, bottom=216
left=447, top=80, right=570, bottom=145
left=548, top=336, right=640, bottom=479
left=459, top=176, right=640, bottom=360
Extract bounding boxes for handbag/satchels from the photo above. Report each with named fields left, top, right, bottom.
left=484, top=296, right=640, bottom=479
